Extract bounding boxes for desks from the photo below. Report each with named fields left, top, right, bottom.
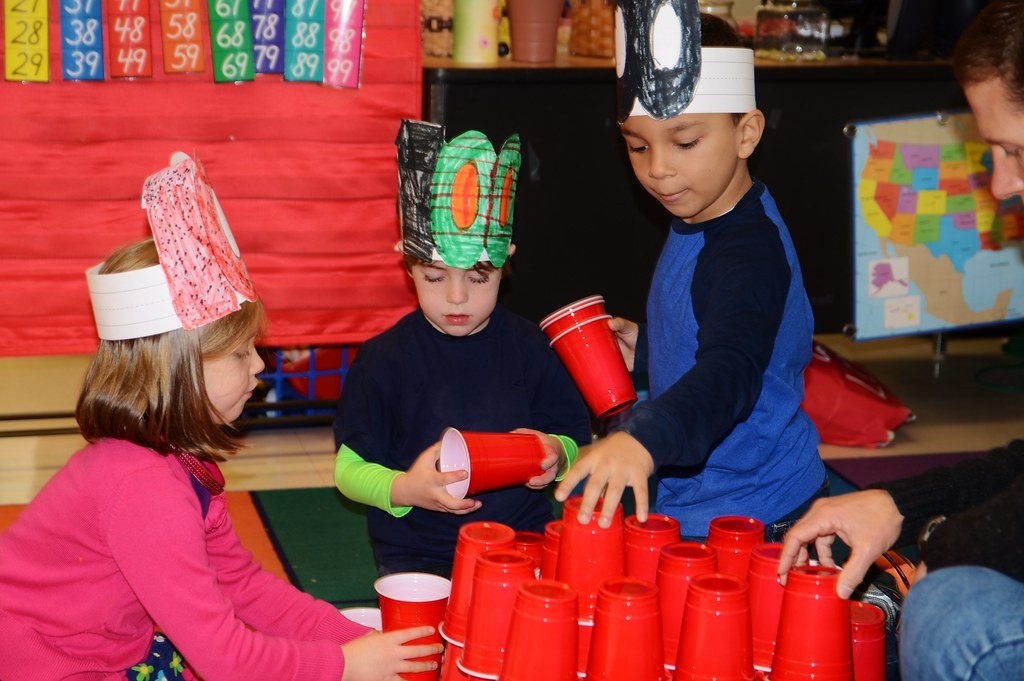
left=426, top=47, right=951, bottom=331
left=0, top=332, right=1024, bottom=588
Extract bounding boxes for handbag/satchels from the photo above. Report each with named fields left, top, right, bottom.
left=800, top=339, right=917, bottom=449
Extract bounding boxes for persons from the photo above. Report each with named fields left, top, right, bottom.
left=554, top=13, right=828, bottom=544
left=331, top=208, right=590, bottom=584
left=775, top=0, right=1024, bottom=681
left=1, top=240, right=446, bottom=681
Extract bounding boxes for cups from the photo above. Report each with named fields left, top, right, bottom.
left=540, top=296, right=639, bottom=416
left=451, top=0, right=503, bottom=67
left=506, top=0, right=564, bottom=63
left=373, top=428, right=885, bottom=681
